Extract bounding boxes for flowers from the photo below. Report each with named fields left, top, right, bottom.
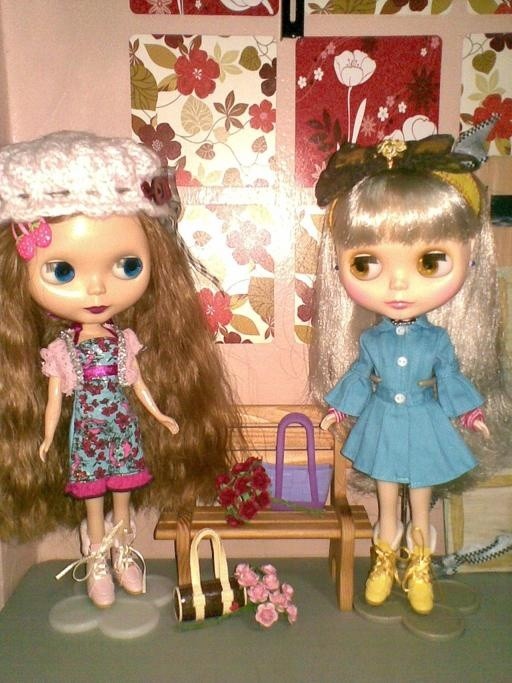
left=231, top=555, right=300, bottom=628
left=214, top=454, right=273, bottom=528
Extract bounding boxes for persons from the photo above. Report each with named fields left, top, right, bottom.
left=307, top=113, right=511, bottom=615
left=1, top=132, right=254, bottom=608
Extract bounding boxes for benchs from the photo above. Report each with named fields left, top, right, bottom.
left=150, top=397, right=382, bottom=613
